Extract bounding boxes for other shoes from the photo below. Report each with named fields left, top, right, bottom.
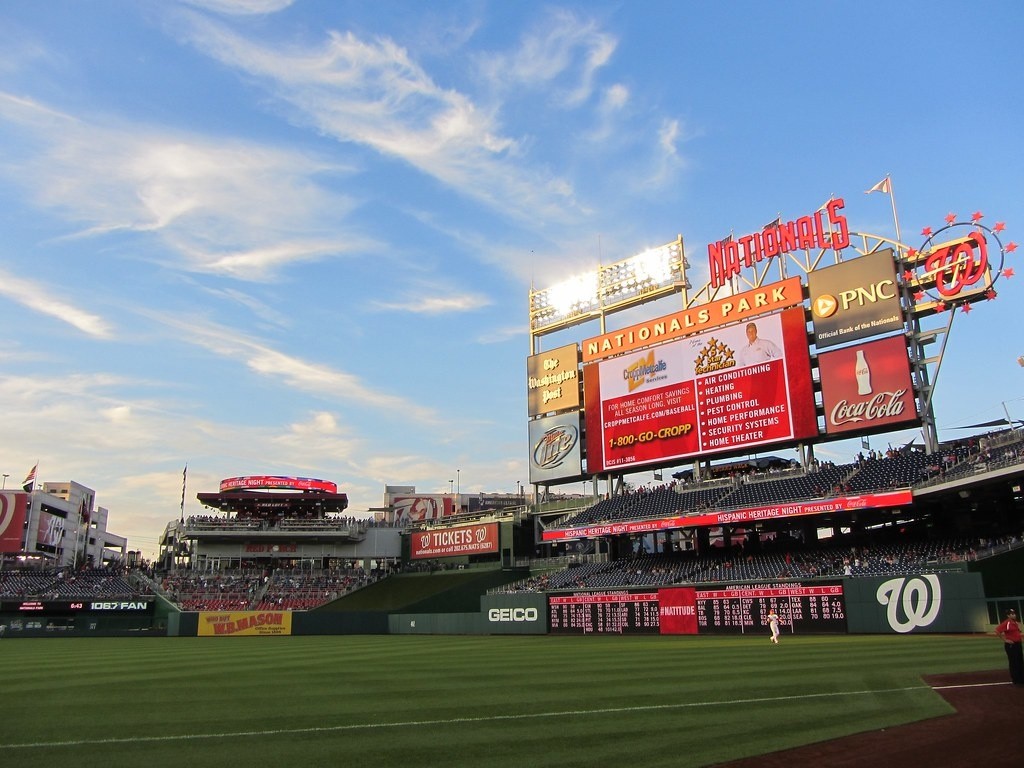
left=770, top=637, right=778, bottom=643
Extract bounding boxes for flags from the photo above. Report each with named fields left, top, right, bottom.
left=179, top=464, right=187, bottom=508
left=20, top=464, right=38, bottom=484
left=23, top=480, right=35, bottom=493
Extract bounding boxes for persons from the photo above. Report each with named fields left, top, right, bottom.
left=498, top=522, right=1024, bottom=605
left=0, top=623, right=7, bottom=639
left=766, top=609, right=784, bottom=643
left=538, top=425, right=1024, bottom=505
left=0, top=556, right=471, bottom=613
left=178, top=508, right=531, bottom=539
left=994, top=609, right=1024, bottom=689
left=739, top=323, right=781, bottom=367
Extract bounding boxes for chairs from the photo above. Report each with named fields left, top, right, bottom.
left=481, top=425, right=1024, bottom=595
left=0, top=514, right=388, bottom=613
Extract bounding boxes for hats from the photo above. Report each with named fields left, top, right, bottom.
left=1006, top=608, right=1016, bottom=614
